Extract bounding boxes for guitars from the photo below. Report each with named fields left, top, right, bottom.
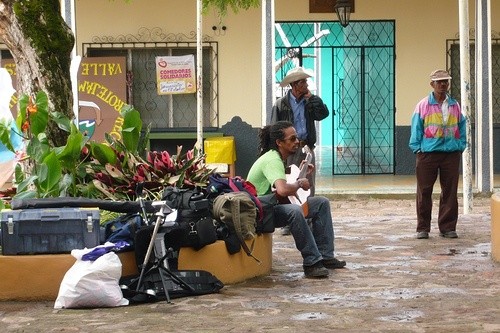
left=276, top=145, right=313, bottom=217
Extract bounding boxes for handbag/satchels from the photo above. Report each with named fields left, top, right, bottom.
left=206, top=173, right=263, bottom=226
left=189, top=216, right=216, bottom=251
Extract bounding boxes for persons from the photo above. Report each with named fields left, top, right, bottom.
left=270, top=68, right=330, bottom=235
left=409, top=70, right=469, bottom=238
left=245, top=122, right=347, bottom=277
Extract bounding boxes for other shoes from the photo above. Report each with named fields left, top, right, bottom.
left=439, top=231, right=456, bottom=237
left=281, top=227, right=291, bottom=235
left=303, top=260, right=329, bottom=277
left=417, top=232, right=429, bottom=238
left=322, top=258, right=346, bottom=267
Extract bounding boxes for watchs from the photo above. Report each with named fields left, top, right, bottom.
left=298, top=179, right=304, bottom=187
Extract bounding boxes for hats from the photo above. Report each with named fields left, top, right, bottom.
left=280, top=67, right=313, bottom=87
left=431, top=69, right=452, bottom=81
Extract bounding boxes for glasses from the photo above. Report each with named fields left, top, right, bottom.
left=282, top=133, right=298, bottom=142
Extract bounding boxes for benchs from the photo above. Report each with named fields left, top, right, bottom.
left=145, top=127, right=225, bottom=163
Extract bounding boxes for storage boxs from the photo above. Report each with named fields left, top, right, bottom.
left=0, top=207, right=100, bottom=255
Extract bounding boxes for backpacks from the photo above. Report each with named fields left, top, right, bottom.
left=215, top=191, right=263, bottom=265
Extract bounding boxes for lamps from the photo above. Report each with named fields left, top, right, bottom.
left=334, top=0, right=351, bottom=28
